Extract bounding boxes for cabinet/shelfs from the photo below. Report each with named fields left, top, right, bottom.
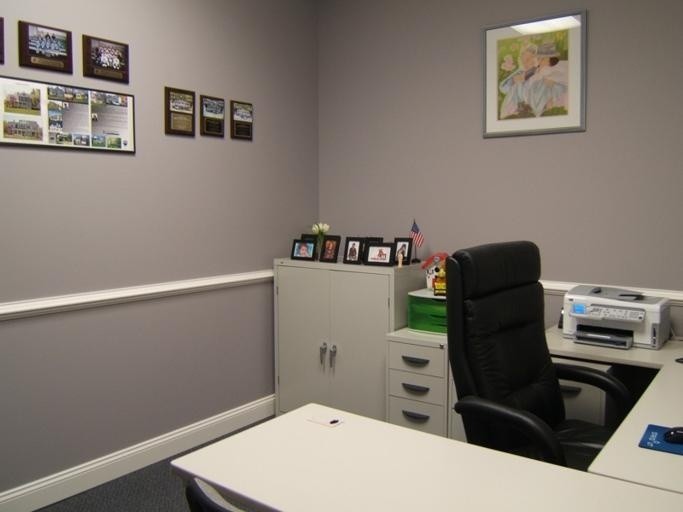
left=273, top=259, right=429, bottom=423
left=385, top=326, right=449, bottom=437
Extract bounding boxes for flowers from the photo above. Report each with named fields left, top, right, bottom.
left=311, top=222, right=329, bottom=243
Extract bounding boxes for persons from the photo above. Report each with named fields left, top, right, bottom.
left=326, top=240, right=334, bottom=258
left=498, top=42, right=539, bottom=114
left=349, top=242, right=356, bottom=260
left=513, top=41, right=569, bottom=114
left=397, top=244, right=406, bottom=256
left=295, top=243, right=310, bottom=257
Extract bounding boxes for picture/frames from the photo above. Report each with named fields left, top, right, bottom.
left=290, top=233, right=412, bottom=266
left=481, top=9, right=588, bottom=138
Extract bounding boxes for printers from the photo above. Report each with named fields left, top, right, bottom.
left=563, top=285, right=669, bottom=350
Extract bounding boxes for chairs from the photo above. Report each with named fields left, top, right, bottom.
left=445, top=239, right=635, bottom=472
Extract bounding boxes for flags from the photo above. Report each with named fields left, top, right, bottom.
left=409, top=222, right=424, bottom=248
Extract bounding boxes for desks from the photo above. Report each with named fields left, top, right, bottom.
left=169, top=320, right=682, bottom=512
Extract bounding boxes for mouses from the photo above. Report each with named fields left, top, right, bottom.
left=664, top=428, right=683, bottom=444
